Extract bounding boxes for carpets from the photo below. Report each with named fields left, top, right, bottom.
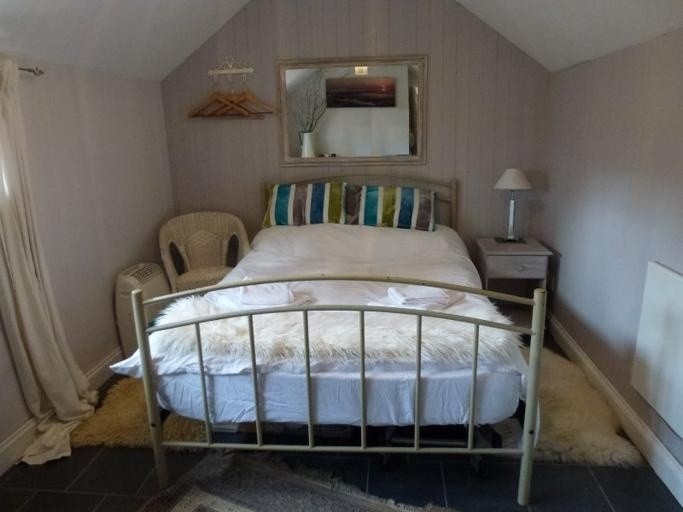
left=135, top=444, right=458, bottom=512
left=476, top=345, right=646, bottom=469
left=70, top=376, right=206, bottom=451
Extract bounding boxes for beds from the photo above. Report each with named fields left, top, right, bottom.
left=131, top=173, right=547, bottom=507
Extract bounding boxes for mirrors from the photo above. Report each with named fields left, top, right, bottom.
left=279, top=54, right=427, bottom=165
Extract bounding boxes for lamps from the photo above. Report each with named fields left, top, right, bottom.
left=493, top=168, right=533, bottom=244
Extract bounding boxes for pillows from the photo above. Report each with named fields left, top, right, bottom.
left=260, top=181, right=437, bottom=232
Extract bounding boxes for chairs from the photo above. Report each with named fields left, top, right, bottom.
left=158, top=212, right=250, bottom=293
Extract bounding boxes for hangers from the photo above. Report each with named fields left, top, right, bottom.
left=187, top=83, right=278, bottom=120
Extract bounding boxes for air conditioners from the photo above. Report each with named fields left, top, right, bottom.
left=115, top=262, right=170, bottom=360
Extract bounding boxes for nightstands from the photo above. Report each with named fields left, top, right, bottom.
left=473, top=238, right=554, bottom=290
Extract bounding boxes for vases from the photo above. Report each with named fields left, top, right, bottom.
left=301, top=133, right=316, bottom=159
